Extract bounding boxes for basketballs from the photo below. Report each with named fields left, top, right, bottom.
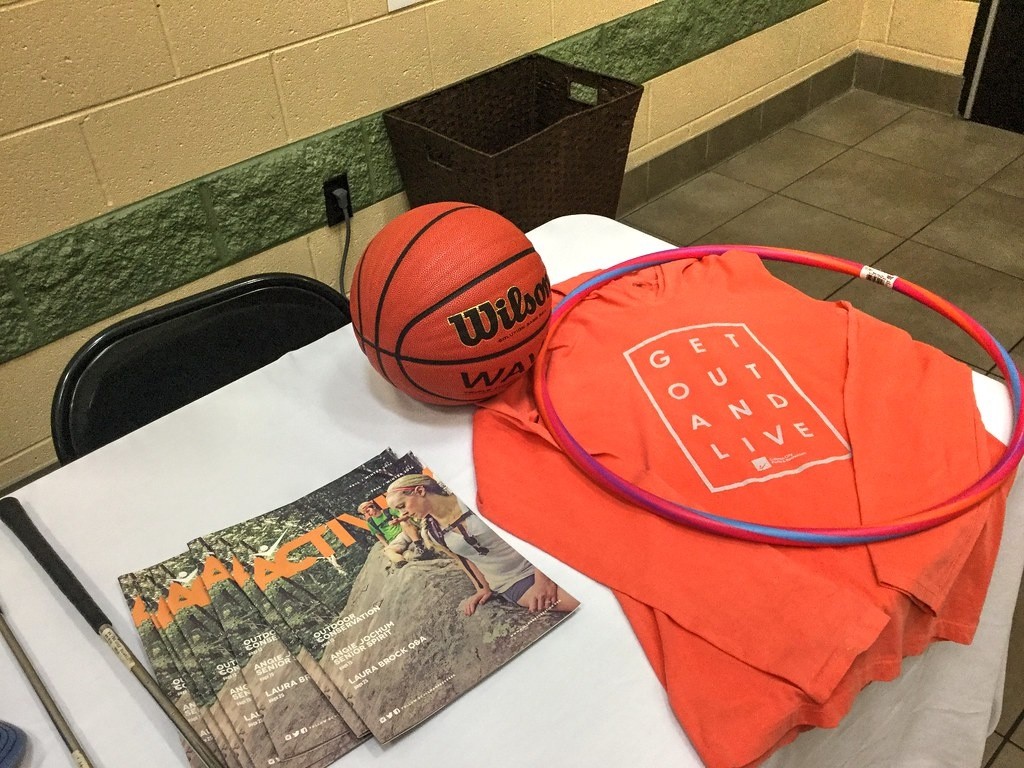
left=349, top=201, right=552, bottom=412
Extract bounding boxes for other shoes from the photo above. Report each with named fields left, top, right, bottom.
left=413, top=545, right=425, bottom=561
left=395, top=560, right=408, bottom=569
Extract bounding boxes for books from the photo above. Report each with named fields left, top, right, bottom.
left=117, top=446, right=581, bottom=768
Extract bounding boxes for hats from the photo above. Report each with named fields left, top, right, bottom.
left=357, top=502, right=374, bottom=514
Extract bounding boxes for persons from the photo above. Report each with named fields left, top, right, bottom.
left=386, top=474, right=581, bottom=617
left=358, top=501, right=425, bottom=568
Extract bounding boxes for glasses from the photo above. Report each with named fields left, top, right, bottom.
left=464, top=537, right=490, bottom=556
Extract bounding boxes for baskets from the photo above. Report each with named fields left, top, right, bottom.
left=384, top=53, right=644, bottom=231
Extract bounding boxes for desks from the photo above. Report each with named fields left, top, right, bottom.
left=0, top=217, right=1024, bottom=768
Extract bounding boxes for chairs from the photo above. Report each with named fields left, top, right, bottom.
left=50, top=271, right=354, bottom=467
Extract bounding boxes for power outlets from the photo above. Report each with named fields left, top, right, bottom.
left=323, top=172, right=353, bottom=228
